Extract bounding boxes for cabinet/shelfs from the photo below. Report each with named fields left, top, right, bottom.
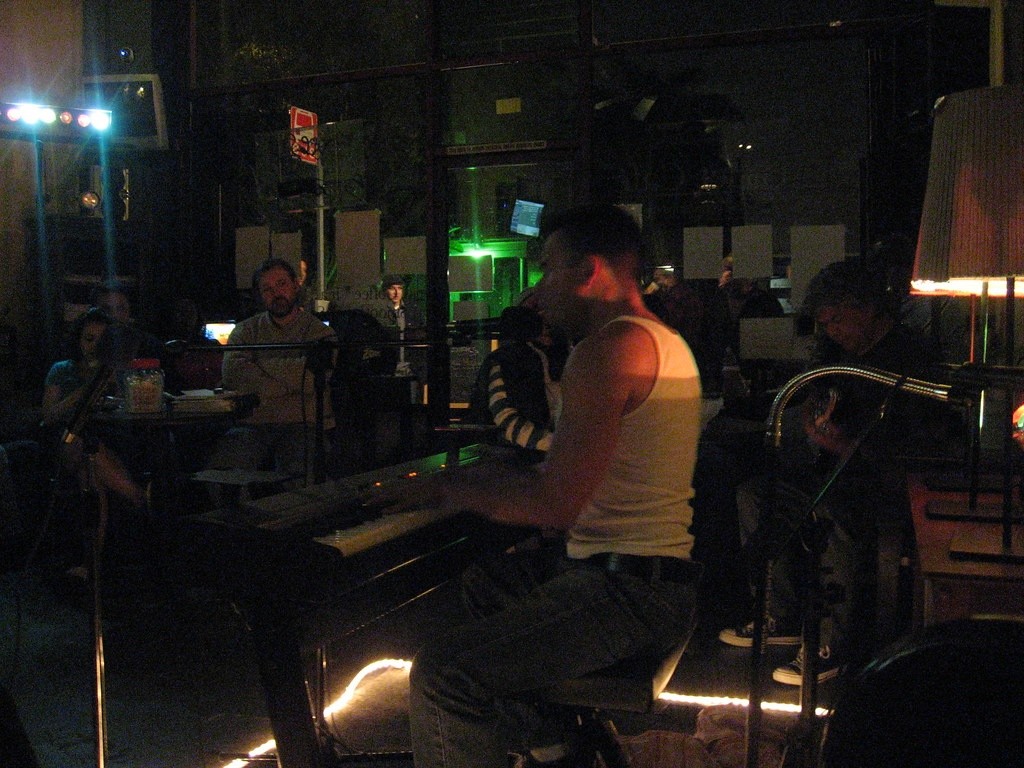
left=902, top=473, right=1024, bottom=631
left=24, top=209, right=152, bottom=364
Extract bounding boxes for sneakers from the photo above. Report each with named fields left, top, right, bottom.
left=772, top=642, right=850, bottom=686
left=719, top=613, right=803, bottom=648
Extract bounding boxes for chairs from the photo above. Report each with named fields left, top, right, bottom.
left=819, top=619, right=1024, bottom=767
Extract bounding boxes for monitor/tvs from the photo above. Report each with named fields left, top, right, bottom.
left=81, top=74, right=170, bottom=151
left=504, top=197, right=549, bottom=241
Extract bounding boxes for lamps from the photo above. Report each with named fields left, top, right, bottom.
left=908, top=85, right=1024, bottom=563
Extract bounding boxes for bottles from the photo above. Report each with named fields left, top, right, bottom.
left=124, top=357, right=166, bottom=414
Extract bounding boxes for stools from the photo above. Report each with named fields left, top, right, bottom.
left=524, top=646, right=684, bottom=768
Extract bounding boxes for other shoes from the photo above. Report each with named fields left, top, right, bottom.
left=506, top=744, right=578, bottom=768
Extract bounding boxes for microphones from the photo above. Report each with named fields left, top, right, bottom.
left=439, top=307, right=543, bottom=338
left=60, top=322, right=142, bottom=445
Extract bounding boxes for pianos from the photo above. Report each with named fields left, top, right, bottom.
left=170, top=440, right=536, bottom=768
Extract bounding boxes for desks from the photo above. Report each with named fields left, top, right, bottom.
left=90, top=405, right=236, bottom=468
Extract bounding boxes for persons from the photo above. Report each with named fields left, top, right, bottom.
left=41, top=276, right=175, bottom=578
left=719, top=259, right=980, bottom=685
left=203, top=257, right=339, bottom=509
left=366, top=203, right=701, bottom=768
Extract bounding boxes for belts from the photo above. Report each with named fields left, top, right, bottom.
left=585, top=553, right=701, bottom=587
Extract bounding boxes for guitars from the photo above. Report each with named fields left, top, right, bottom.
left=798, top=389, right=853, bottom=482
